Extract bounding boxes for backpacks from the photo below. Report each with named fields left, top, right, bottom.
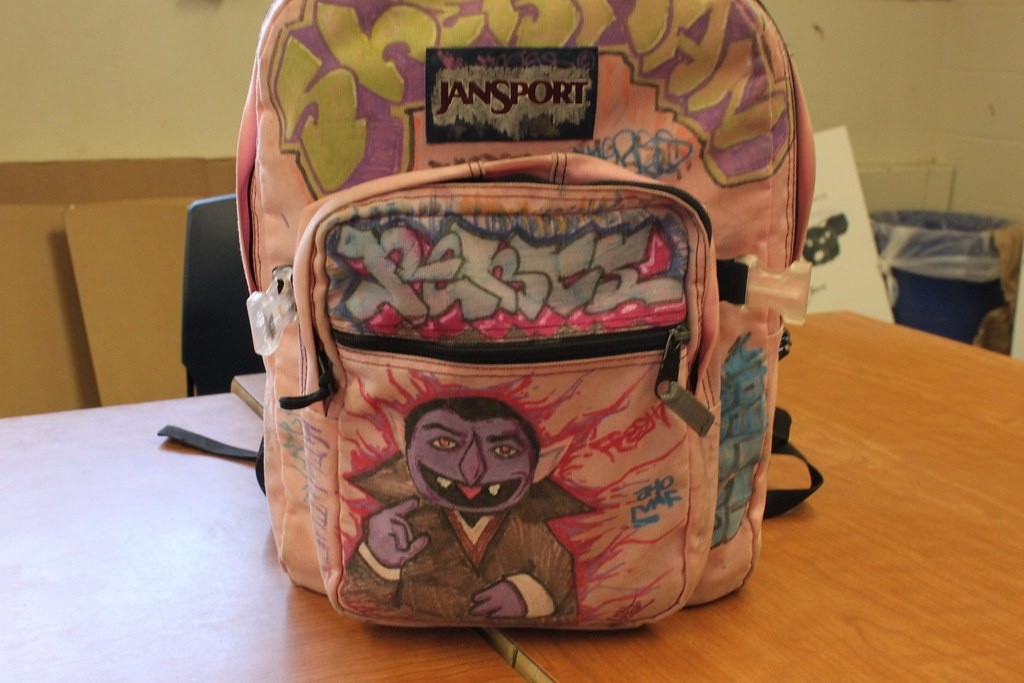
left=154, top=0, right=827, bottom=630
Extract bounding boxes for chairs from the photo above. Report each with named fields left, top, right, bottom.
left=181, top=193, right=266, bottom=397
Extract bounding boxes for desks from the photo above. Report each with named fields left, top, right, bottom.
left=0, top=310, right=1024, bottom=683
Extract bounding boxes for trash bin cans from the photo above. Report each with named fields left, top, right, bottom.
left=868, top=207, right=1024, bottom=343
